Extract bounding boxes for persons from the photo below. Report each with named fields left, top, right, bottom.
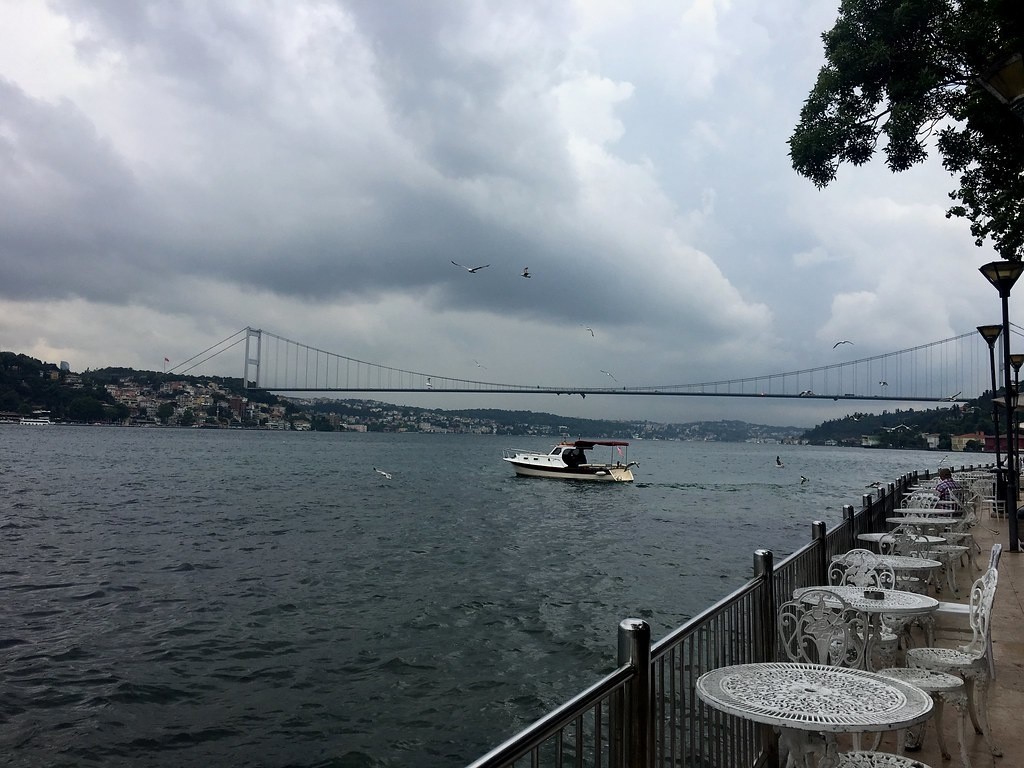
left=931, top=467, right=966, bottom=517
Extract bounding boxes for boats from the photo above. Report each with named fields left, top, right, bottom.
left=502, top=433, right=641, bottom=483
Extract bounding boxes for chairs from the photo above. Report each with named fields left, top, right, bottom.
left=777, top=473, right=1008, bottom=768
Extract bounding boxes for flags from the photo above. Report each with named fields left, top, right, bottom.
left=616, top=445, right=624, bottom=457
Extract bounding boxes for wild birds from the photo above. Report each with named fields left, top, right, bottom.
left=373, top=467, right=392, bottom=480
left=865, top=481, right=884, bottom=490
left=775, top=455, right=782, bottom=465
left=832, top=339, right=854, bottom=349
left=879, top=381, right=888, bottom=386
left=521, top=266, right=531, bottom=279
left=581, top=322, right=595, bottom=338
left=798, top=390, right=812, bottom=397
left=599, top=369, right=617, bottom=382
left=799, top=476, right=810, bottom=484
left=474, top=359, right=490, bottom=370
left=452, top=261, right=491, bottom=274
left=937, top=391, right=962, bottom=403
left=624, top=460, right=640, bottom=473
left=425, top=377, right=432, bottom=387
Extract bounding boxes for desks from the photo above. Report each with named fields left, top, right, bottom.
left=793, top=586, right=940, bottom=670
left=857, top=534, right=946, bottom=557
left=694, top=663, right=935, bottom=768
left=831, top=554, right=942, bottom=590
left=893, top=508, right=956, bottom=518
left=886, top=517, right=958, bottom=536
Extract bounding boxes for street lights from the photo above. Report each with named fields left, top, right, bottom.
left=979, top=260, right=1024, bottom=552
left=976, top=324, right=1005, bottom=513
left=1008, top=353, right=1024, bottom=502
